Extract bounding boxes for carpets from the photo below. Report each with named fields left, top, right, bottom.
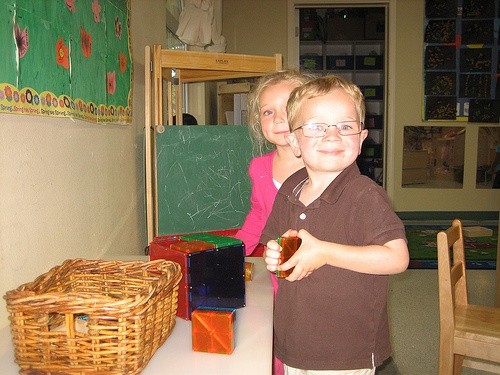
left=404, top=225, right=498, bottom=270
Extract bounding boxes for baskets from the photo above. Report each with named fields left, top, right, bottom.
left=3, top=257, right=183, bottom=375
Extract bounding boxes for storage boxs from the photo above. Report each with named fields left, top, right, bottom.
left=326, top=9, right=385, bottom=39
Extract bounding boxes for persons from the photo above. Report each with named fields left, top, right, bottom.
left=234, top=68, right=319, bottom=375
left=259, top=78, right=410, bottom=375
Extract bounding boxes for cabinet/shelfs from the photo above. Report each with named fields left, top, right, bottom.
left=300, top=39, right=385, bottom=185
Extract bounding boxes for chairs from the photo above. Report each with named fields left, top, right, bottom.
left=437, top=219, right=500, bottom=375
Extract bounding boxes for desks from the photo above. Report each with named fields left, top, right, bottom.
left=0, top=255, right=274, bottom=375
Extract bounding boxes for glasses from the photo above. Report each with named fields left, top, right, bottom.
left=292, top=121, right=364, bottom=137
